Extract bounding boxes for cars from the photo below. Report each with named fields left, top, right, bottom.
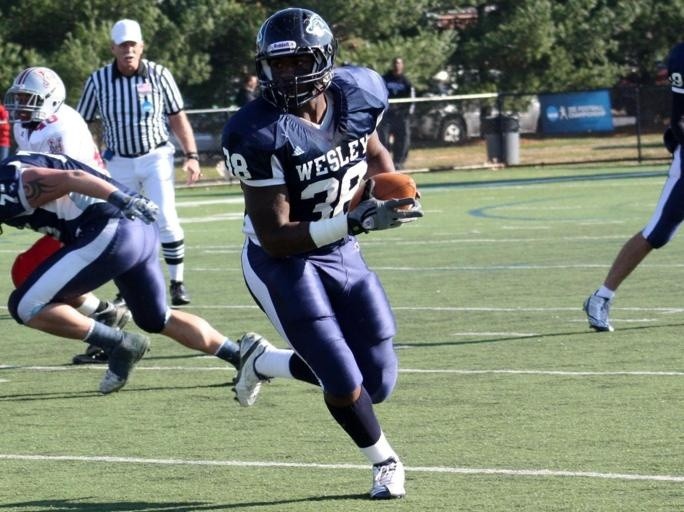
left=412, top=70, right=541, bottom=144
left=611, top=59, right=672, bottom=116
left=163, top=109, right=226, bottom=163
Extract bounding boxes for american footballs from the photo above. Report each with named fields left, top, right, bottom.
left=348, top=172, right=416, bottom=212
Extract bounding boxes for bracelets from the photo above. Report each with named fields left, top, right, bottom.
left=183, top=151, right=200, bottom=160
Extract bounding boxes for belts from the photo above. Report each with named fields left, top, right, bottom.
left=120, top=141, right=166, bottom=158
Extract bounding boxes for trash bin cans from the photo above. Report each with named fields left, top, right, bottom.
left=484, top=116, right=520, bottom=166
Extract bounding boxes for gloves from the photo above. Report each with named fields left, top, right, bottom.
left=344, top=180, right=421, bottom=236
left=120, top=196, right=158, bottom=226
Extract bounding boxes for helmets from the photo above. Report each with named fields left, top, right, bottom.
left=254, top=7, right=336, bottom=112
left=0, top=67, right=67, bottom=125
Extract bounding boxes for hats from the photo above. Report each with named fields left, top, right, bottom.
left=111, top=19, right=142, bottom=45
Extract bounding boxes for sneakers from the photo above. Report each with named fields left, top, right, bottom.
left=113, top=294, right=127, bottom=307
left=86, top=301, right=131, bottom=358
left=370, top=454, right=405, bottom=499
left=99, top=329, right=151, bottom=394
left=169, top=280, right=190, bottom=306
left=583, top=290, right=614, bottom=332
left=232, top=332, right=273, bottom=408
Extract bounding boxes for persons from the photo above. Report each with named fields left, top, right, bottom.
left=581, top=43, right=684, bottom=333
left=0, top=103, right=10, bottom=162
left=376, top=56, right=416, bottom=170
left=236, top=73, right=259, bottom=106
left=3, top=67, right=131, bottom=365
left=0, top=149, right=241, bottom=395
left=76, top=18, right=201, bottom=308
left=219, top=7, right=426, bottom=500
